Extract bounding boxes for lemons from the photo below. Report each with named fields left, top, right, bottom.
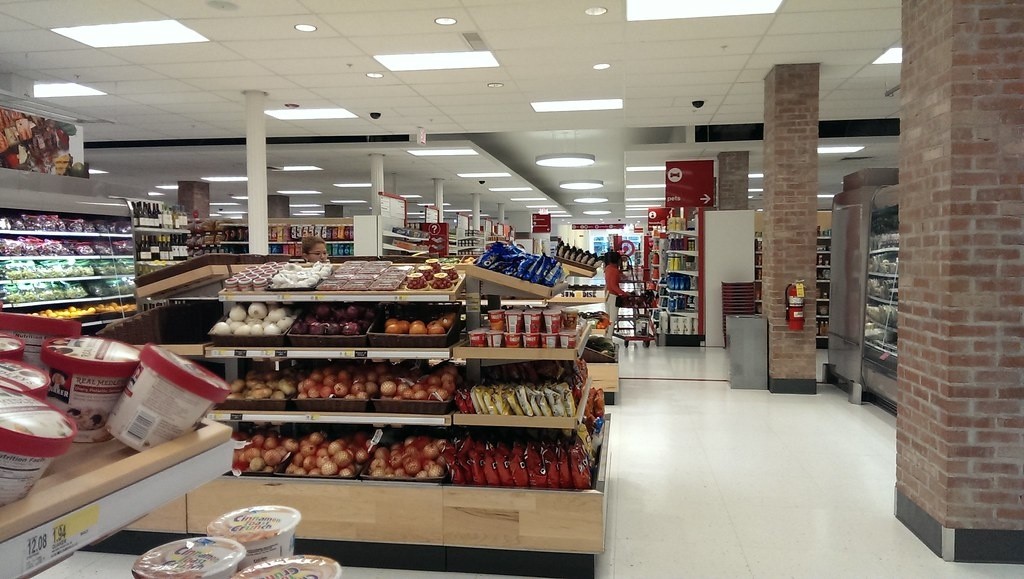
left=26, top=301, right=135, bottom=318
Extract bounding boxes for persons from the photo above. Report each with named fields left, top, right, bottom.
left=603, top=247, right=631, bottom=343
left=302, top=236, right=327, bottom=262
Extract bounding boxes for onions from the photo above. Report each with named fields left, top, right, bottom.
left=208, top=303, right=457, bottom=478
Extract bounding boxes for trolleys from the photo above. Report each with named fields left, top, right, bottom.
left=613, top=280, right=657, bottom=348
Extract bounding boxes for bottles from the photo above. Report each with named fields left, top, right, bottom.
left=816, top=319, right=828, bottom=336
left=131, top=200, right=187, bottom=229
left=135, top=233, right=188, bottom=261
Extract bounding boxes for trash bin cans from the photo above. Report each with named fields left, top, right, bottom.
left=725, top=313, right=768, bottom=391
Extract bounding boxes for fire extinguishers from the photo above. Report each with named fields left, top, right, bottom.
left=785, top=278, right=806, bottom=330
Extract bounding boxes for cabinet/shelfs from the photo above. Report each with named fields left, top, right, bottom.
left=0, top=230, right=135, bottom=328
left=78, top=196, right=831, bottom=555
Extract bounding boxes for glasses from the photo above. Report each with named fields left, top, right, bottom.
left=309, top=251, right=327, bottom=256
